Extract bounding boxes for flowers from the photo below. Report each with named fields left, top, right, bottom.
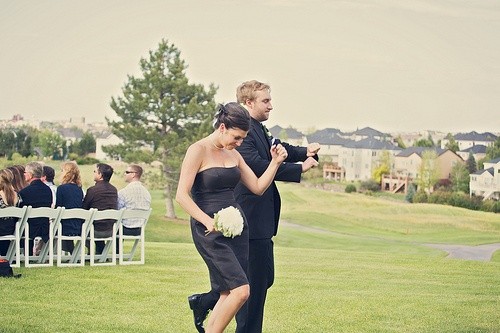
left=204, top=205, right=244, bottom=239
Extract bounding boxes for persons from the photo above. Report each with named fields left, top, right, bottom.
left=0, top=168, right=23, bottom=276
left=81, top=163, right=118, bottom=255
left=174, top=102, right=288, bottom=333
left=117, top=163, right=152, bottom=254
left=188, top=81, right=321, bottom=333
left=6, top=160, right=58, bottom=263
left=54, top=163, right=83, bottom=263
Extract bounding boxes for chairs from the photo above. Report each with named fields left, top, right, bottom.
left=74, top=208, right=125, bottom=268
left=10, top=205, right=61, bottom=268
left=0, top=204, right=27, bottom=270
left=43, top=207, right=94, bottom=266
left=103, top=207, right=153, bottom=268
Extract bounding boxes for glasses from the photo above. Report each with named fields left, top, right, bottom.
left=23, top=170, right=28, bottom=174
left=124, top=170, right=138, bottom=174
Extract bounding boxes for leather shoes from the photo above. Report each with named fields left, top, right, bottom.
left=188, top=294, right=210, bottom=333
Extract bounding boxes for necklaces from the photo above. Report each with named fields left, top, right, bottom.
left=209, top=134, right=225, bottom=150
